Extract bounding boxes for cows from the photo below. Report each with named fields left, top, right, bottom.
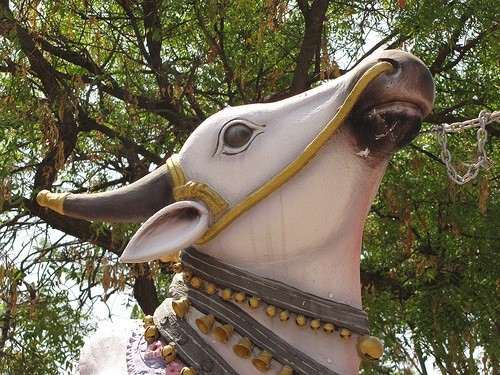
left=34, top=48, right=437, bottom=375
